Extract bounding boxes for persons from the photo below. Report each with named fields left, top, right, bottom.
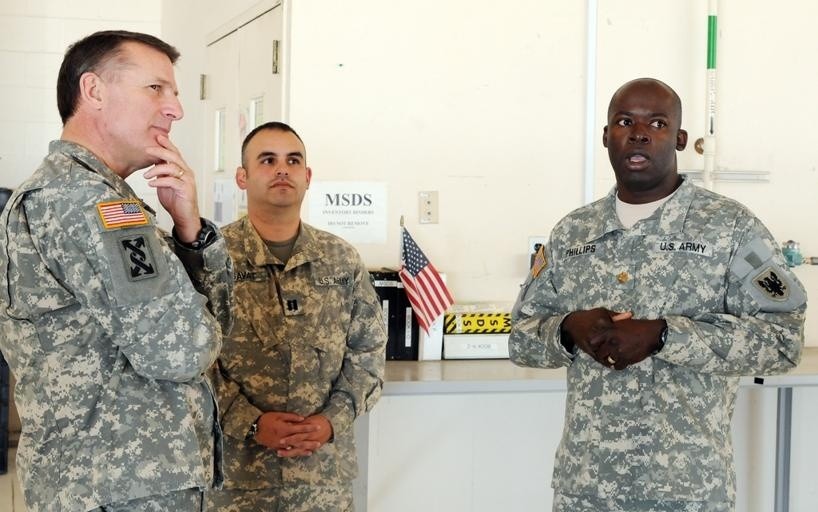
left=507, top=77, right=809, bottom=512
left=196, top=122, right=389, bottom=512
left=0, top=30, right=234, bottom=511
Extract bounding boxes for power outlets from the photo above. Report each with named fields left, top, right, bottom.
left=418, top=191, right=439, bottom=224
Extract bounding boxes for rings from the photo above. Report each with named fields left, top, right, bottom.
left=177, top=167, right=185, bottom=178
left=285, top=446, right=291, bottom=449
left=607, top=355, right=616, bottom=364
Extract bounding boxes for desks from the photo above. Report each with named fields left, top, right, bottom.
left=353, top=346, right=818, bottom=512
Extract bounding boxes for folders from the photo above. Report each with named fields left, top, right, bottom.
left=369, top=271, right=418, bottom=361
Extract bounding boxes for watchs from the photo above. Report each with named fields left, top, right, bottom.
left=248, top=421, right=258, bottom=438
left=184, top=226, right=214, bottom=247
left=660, top=325, right=669, bottom=354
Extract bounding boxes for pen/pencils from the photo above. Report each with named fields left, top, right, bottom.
left=611, top=311, right=634, bottom=322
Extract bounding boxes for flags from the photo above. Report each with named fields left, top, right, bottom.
left=399, top=227, right=454, bottom=337
